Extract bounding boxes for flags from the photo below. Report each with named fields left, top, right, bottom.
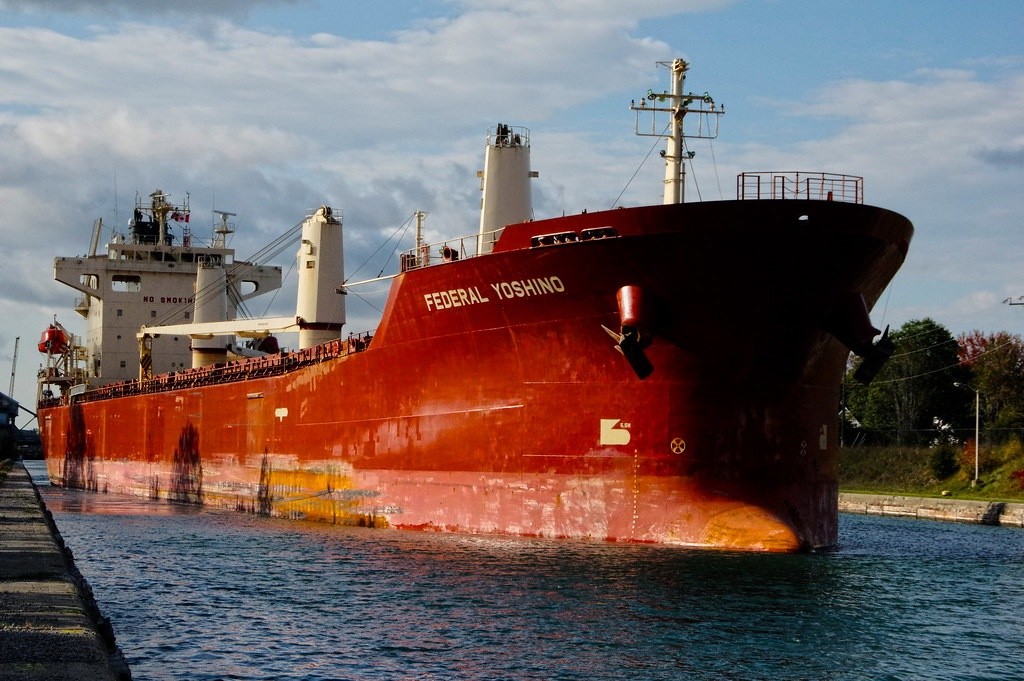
left=172, top=212, right=189, bottom=224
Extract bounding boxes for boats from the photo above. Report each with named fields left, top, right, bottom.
left=32, top=57, right=916, bottom=554
